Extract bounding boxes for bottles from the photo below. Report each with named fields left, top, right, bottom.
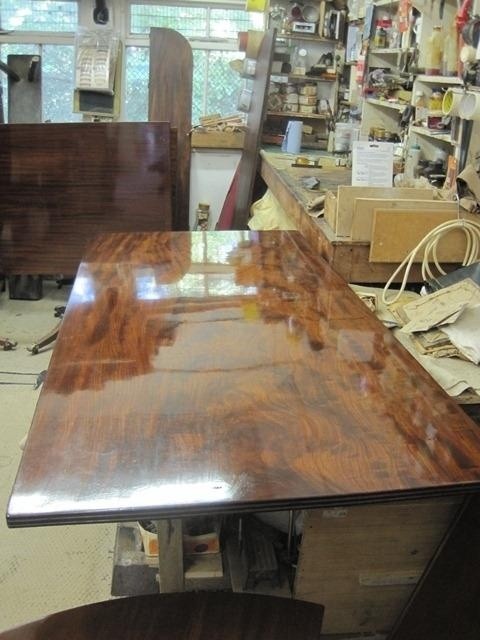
left=442, top=24, right=457, bottom=75
left=429, top=88, right=443, bottom=112
left=375, top=26, right=385, bottom=49
left=368, top=127, right=385, bottom=143
left=339, top=76, right=346, bottom=98
left=343, top=89, right=350, bottom=101
left=425, top=25, right=442, bottom=74
left=405, top=146, right=422, bottom=177
left=432, top=147, right=448, bottom=168
left=414, top=91, right=426, bottom=125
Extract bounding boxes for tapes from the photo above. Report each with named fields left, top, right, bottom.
left=441, top=87, right=480, bottom=121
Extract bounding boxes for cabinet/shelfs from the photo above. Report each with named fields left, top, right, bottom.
left=337, top=15, right=370, bottom=113
left=405, top=73, right=466, bottom=146
left=264, top=0, right=343, bottom=122
left=366, top=0, right=416, bottom=117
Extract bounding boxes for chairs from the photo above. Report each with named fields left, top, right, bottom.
left=0, top=578, right=328, bottom=639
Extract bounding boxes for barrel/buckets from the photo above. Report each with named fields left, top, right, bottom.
left=334, top=122, right=356, bottom=152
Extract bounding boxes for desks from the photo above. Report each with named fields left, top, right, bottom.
left=5, top=226, right=479, bottom=594
left=258, top=144, right=439, bottom=292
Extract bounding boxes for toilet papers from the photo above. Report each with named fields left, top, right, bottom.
left=279, top=120, right=303, bottom=155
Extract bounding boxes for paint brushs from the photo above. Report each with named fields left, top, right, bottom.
left=326, top=99, right=336, bottom=128
